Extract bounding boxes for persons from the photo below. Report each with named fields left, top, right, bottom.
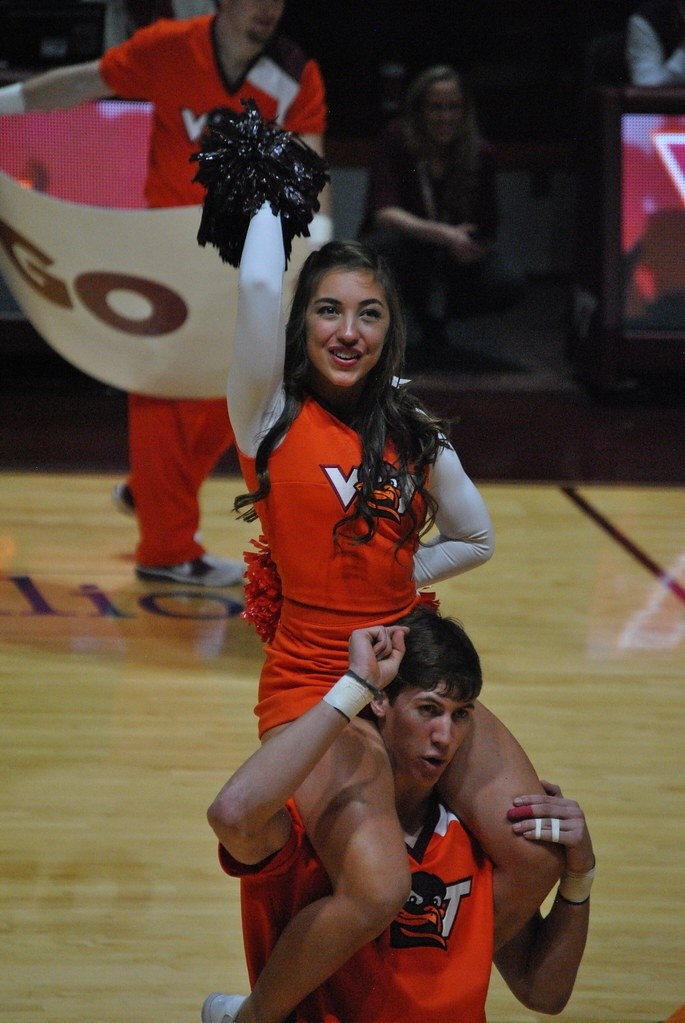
left=187, top=95, right=596, bottom=1023
left=355, top=66, right=530, bottom=376
left=0, top=0, right=332, bottom=591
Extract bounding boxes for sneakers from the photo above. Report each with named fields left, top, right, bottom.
left=110, top=480, right=139, bottom=516
left=201, top=990, right=247, bottom=1023
left=137, top=543, right=243, bottom=585
